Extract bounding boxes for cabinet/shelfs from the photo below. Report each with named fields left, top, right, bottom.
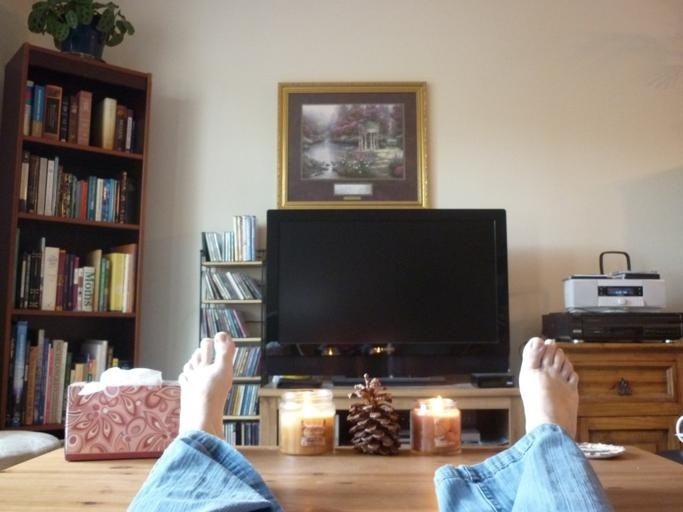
left=554, top=339, right=681, bottom=452
left=0, top=40, right=155, bottom=443
left=198, top=244, right=264, bottom=449
left=256, top=384, right=529, bottom=455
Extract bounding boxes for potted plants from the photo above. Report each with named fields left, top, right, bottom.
left=25, top=1, right=133, bottom=61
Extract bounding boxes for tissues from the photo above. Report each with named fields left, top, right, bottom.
left=64, top=366, right=181, bottom=462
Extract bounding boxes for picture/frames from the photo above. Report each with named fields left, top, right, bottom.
left=274, top=79, right=429, bottom=212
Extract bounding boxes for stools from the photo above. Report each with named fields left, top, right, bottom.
left=0, top=429, right=60, bottom=471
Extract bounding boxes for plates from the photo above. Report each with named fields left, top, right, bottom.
left=576, top=441, right=625, bottom=459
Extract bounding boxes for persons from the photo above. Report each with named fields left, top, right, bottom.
left=124, top=332, right=613, bottom=512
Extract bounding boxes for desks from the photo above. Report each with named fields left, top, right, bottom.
left=0, top=438, right=683, bottom=511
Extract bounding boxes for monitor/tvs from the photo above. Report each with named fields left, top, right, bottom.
left=263, top=209, right=509, bottom=385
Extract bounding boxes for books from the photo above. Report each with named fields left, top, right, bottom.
left=201, top=215, right=263, bottom=445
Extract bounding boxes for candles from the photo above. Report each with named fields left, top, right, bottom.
left=409, top=396, right=460, bottom=455
left=281, top=392, right=334, bottom=452
